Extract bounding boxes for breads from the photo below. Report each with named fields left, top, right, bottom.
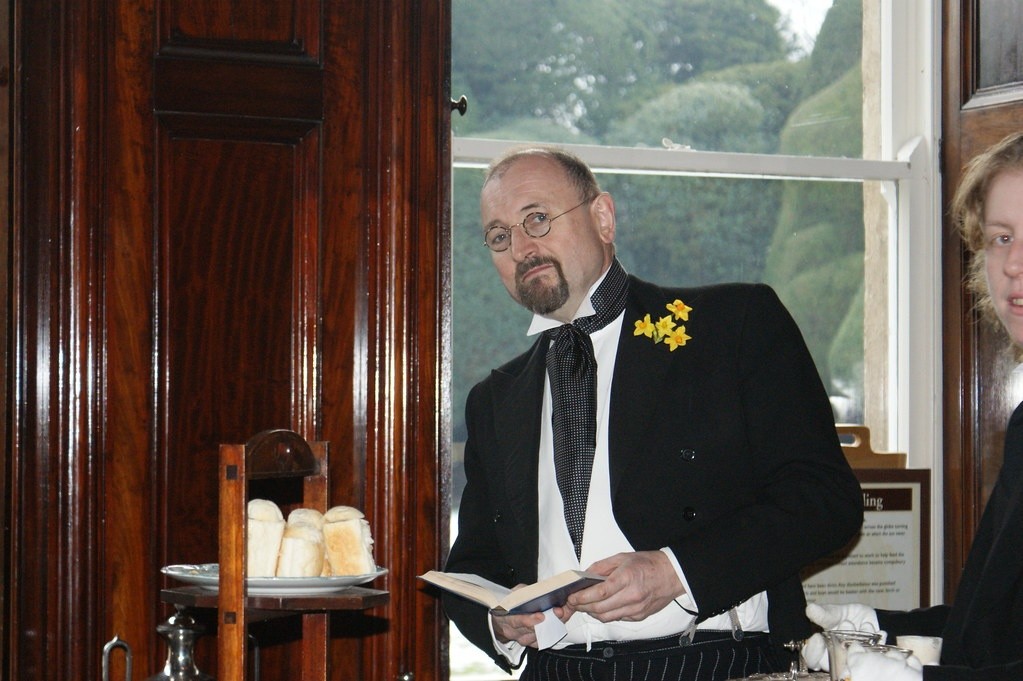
left=245, top=497, right=376, bottom=579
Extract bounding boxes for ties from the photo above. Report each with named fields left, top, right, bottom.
left=544, top=256, right=662, bottom=563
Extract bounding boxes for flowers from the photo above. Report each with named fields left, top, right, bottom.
left=634, top=300, right=691, bottom=351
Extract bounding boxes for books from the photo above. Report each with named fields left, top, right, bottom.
left=417, top=570, right=608, bottom=614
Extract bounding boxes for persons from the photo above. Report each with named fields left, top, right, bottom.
left=437, top=149, right=866, bottom=681
left=801, top=133, right=1023, bottom=681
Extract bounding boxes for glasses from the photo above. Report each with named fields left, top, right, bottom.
left=481, top=192, right=599, bottom=254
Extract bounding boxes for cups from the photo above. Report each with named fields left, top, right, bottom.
left=820, top=631, right=943, bottom=681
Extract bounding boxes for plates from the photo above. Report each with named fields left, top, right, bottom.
left=161, top=562, right=389, bottom=596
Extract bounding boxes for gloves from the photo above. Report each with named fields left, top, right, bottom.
left=801, top=602, right=887, bottom=672
left=845, top=643, right=923, bottom=681
